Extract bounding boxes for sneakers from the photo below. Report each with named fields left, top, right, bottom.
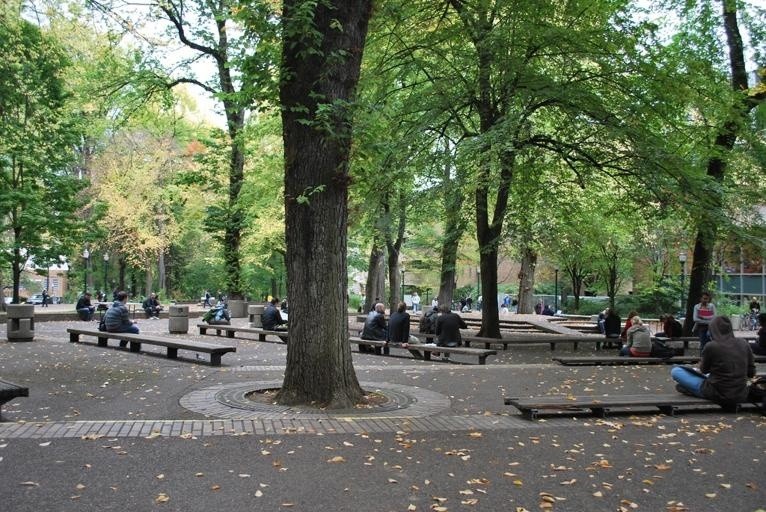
left=431, top=352, right=441, bottom=358
left=442, top=358, right=449, bottom=362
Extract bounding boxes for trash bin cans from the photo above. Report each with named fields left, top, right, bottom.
left=732, top=314, right=740, bottom=330
left=169, top=305, right=189, bottom=333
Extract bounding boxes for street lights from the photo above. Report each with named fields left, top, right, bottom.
left=101, top=251, right=109, bottom=300
left=51, top=283, right=55, bottom=292
left=81, top=248, right=89, bottom=295
left=677, top=248, right=687, bottom=319
left=399, top=261, right=560, bottom=315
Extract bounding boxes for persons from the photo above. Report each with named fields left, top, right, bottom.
left=260, top=297, right=289, bottom=343
left=360, top=302, right=390, bottom=356
left=216, top=289, right=223, bottom=305
left=203, top=288, right=212, bottom=309
left=142, top=292, right=161, bottom=320
left=542, top=304, right=554, bottom=315
left=621, top=311, right=639, bottom=337
left=112, top=287, right=119, bottom=303
left=605, top=309, right=621, bottom=348
left=598, top=307, right=610, bottom=348
left=102, top=290, right=139, bottom=348
left=431, top=292, right=517, bottom=314
left=75, top=292, right=95, bottom=321
left=655, top=316, right=669, bottom=337
left=432, top=303, right=468, bottom=361
left=388, top=300, right=422, bottom=358
left=411, top=292, right=421, bottom=313
left=748, top=313, right=766, bottom=356
left=533, top=298, right=545, bottom=314
left=358, top=293, right=366, bottom=313
left=280, top=296, right=288, bottom=314
left=418, top=305, right=439, bottom=334
left=370, top=297, right=379, bottom=311
left=669, top=315, right=683, bottom=337
left=41, top=290, right=48, bottom=307
left=749, top=296, right=760, bottom=330
left=206, top=303, right=231, bottom=336
left=669, top=315, right=757, bottom=407
left=691, top=292, right=716, bottom=355
left=618, top=316, right=653, bottom=357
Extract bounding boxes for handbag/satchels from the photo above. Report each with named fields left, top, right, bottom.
left=99, top=322, right=106, bottom=331
left=747, top=376, right=766, bottom=402
left=97, top=304, right=108, bottom=311
left=360, top=344, right=375, bottom=353
left=652, top=341, right=675, bottom=358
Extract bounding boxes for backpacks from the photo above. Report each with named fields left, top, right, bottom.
left=419, top=311, right=434, bottom=332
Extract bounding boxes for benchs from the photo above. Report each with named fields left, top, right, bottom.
left=64, top=324, right=236, bottom=365
left=498, top=388, right=720, bottom=421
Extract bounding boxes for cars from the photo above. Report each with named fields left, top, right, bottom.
left=4, top=293, right=67, bottom=307
